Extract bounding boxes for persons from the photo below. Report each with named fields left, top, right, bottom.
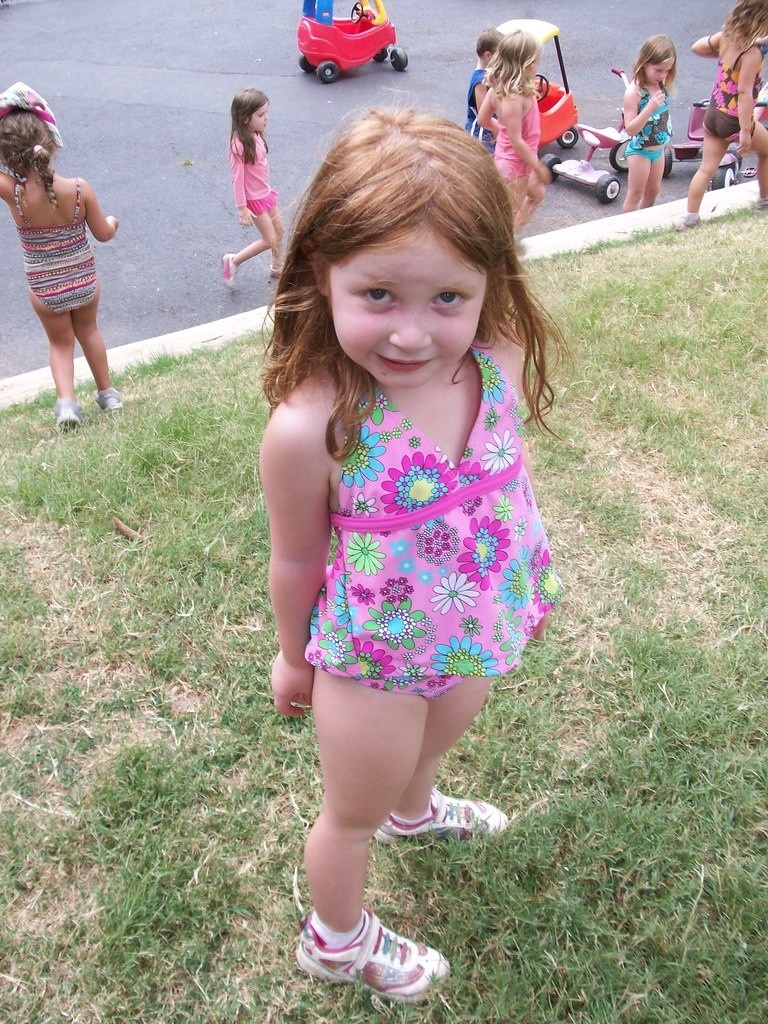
left=0, top=82, right=120, bottom=428
left=478, top=28, right=552, bottom=256
left=465, top=28, right=504, bottom=159
left=222, top=88, right=286, bottom=288
left=255, top=107, right=565, bottom=1002
left=679, top=0, right=768, bottom=230
left=623, top=34, right=676, bottom=213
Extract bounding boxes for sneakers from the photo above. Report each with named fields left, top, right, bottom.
left=96, top=387, right=123, bottom=411
left=375, top=786, right=511, bottom=846
left=296, top=905, right=450, bottom=1002
left=222, top=254, right=240, bottom=289
left=271, top=266, right=282, bottom=278
left=54, top=398, right=83, bottom=428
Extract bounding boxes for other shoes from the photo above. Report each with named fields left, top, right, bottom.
left=756, top=198, right=768, bottom=211
left=683, top=217, right=702, bottom=229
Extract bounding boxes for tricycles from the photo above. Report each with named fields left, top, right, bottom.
left=672, top=98, right=768, bottom=192
left=540, top=66, right=630, bottom=204
